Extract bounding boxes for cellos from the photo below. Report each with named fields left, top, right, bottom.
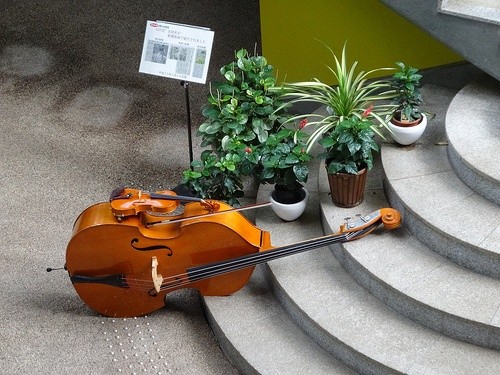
left=62, top=187, right=404, bottom=319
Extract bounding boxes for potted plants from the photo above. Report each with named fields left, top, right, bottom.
left=256, top=120, right=309, bottom=222
left=269, top=36, right=382, bottom=208
left=191, top=46, right=283, bottom=199
left=380, top=60, right=429, bottom=147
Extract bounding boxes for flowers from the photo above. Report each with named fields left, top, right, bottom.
left=350, top=105, right=374, bottom=117
left=290, top=117, right=307, bottom=130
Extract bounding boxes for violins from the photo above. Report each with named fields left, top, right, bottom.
left=107, top=186, right=220, bottom=217
